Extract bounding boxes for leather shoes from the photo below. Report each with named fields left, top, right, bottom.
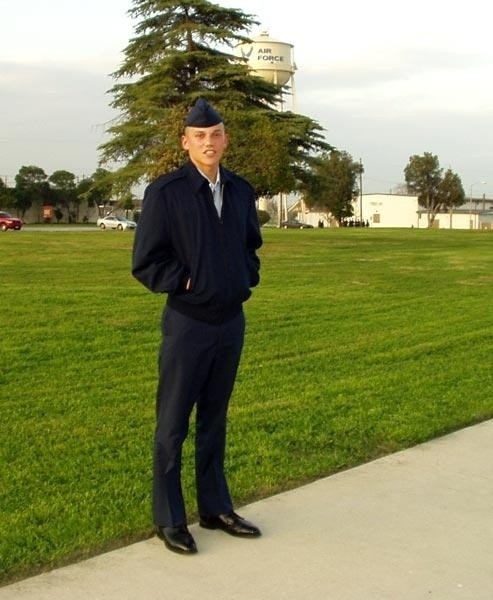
left=200, top=512, right=261, bottom=537
left=156, top=526, right=197, bottom=554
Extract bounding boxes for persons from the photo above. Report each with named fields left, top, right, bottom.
left=129, top=97, right=265, bottom=555
left=317, top=218, right=370, bottom=228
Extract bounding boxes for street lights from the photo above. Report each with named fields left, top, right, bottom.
left=469, top=180, right=488, bottom=229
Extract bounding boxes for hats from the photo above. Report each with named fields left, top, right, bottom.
left=185, top=99, right=221, bottom=128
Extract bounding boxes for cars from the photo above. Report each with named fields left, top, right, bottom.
left=0, top=210, right=23, bottom=233
left=282, top=219, right=314, bottom=230
left=96, top=214, right=138, bottom=231
